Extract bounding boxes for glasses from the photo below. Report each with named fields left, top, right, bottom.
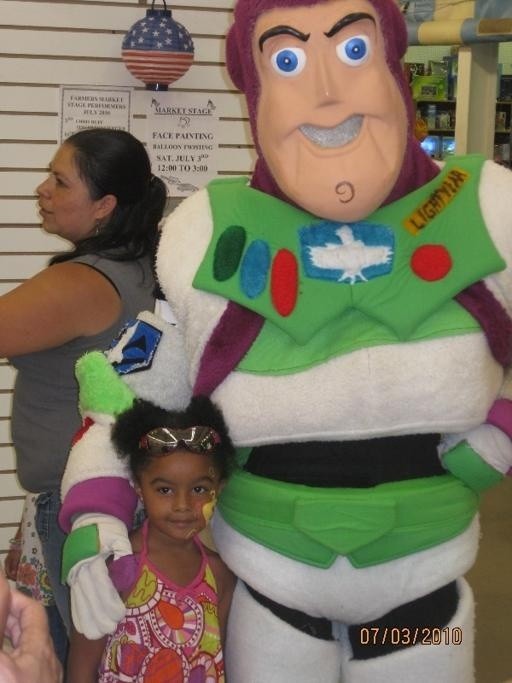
left=135, top=423, right=224, bottom=458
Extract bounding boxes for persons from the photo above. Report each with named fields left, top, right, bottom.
left=2, top=125, right=165, bottom=649
left=58, top=0, right=512, bottom=683
left=1, top=567, right=63, bottom=683
left=45, top=397, right=234, bottom=681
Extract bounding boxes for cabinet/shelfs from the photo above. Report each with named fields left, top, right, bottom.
left=410, top=98, right=512, bottom=167
